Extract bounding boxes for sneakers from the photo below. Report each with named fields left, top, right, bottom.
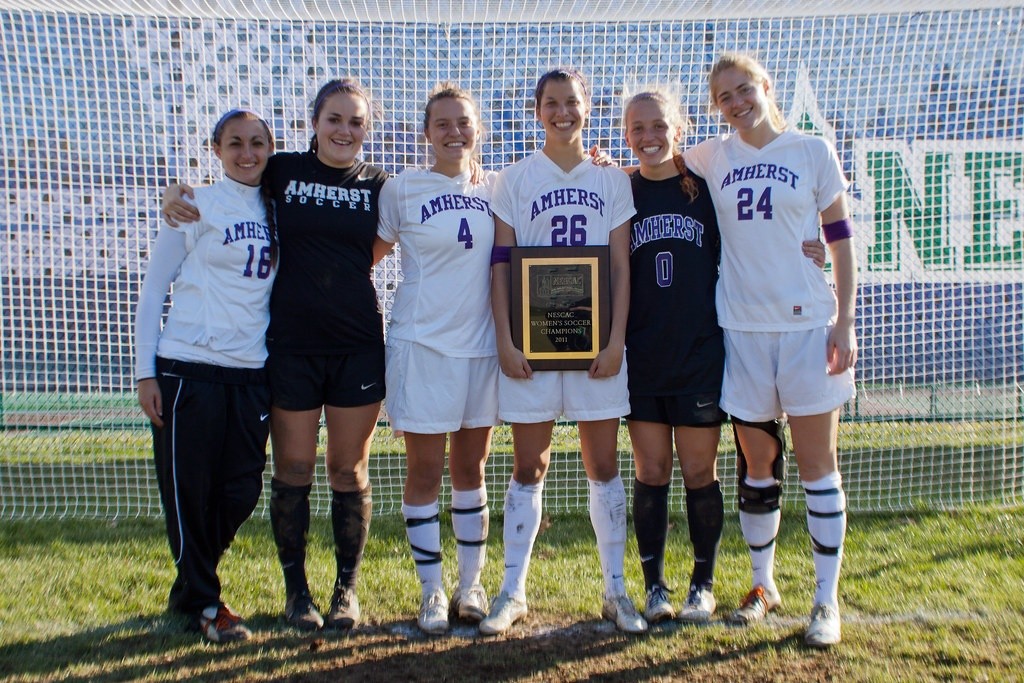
left=644, top=583, right=676, bottom=623
left=199, top=603, right=252, bottom=645
left=284, top=597, right=325, bottom=630
left=450, top=586, right=486, bottom=624
left=328, top=584, right=361, bottom=629
left=805, top=601, right=841, bottom=647
left=677, top=582, right=717, bottom=623
left=479, top=589, right=528, bottom=635
left=731, top=586, right=782, bottom=624
left=603, top=598, right=648, bottom=633
left=418, top=590, right=449, bottom=635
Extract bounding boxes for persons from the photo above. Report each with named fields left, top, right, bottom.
left=370, top=80, right=612, bottom=634
left=623, top=91, right=825, bottom=622
left=617, top=53, right=857, bottom=646
left=481, top=68, right=649, bottom=635
left=133, top=107, right=279, bottom=643
left=163, top=77, right=484, bottom=632
